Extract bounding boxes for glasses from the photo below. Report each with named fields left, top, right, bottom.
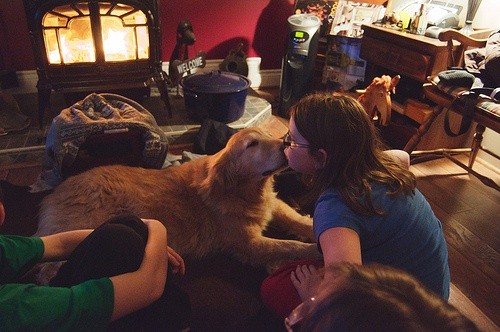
left=283, top=132, right=328, bottom=153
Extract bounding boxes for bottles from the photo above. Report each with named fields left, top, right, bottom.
left=407, top=4, right=429, bottom=35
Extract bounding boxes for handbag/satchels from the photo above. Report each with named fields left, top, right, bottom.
left=426, top=69, right=484, bottom=98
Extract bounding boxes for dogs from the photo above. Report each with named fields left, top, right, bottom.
left=28, top=128, right=316, bottom=281
left=168, top=20, right=196, bottom=88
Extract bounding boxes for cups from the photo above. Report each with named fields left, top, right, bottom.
left=288, top=11, right=321, bottom=56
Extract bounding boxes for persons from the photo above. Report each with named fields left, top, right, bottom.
left=0, top=202, right=190, bottom=332
left=259, top=92, right=450, bottom=320
left=284, top=262, right=466, bottom=332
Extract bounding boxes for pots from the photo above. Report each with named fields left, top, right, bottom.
left=179, top=68, right=251, bottom=124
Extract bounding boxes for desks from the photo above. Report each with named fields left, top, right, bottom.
left=358, top=23, right=468, bottom=101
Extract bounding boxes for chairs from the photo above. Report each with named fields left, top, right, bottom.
left=403, top=28, right=500, bottom=192
left=0, top=90, right=33, bottom=162
